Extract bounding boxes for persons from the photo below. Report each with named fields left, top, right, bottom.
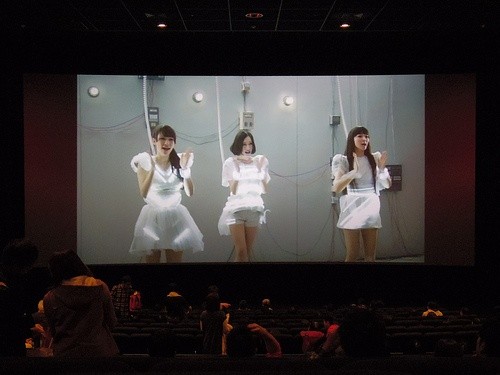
left=198, top=294, right=225, bottom=353
left=332, top=127, right=392, bottom=262
left=301, top=301, right=500, bottom=360
left=111, top=276, right=133, bottom=318
left=43, top=248, right=117, bottom=358
left=222, top=314, right=281, bottom=354
left=217, top=130, right=272, bottom=262
left=163, top=284, right=186, bottom=317
left=0, top=282, right=47, bottom=358
left=129, top=125, right=204, bottom=263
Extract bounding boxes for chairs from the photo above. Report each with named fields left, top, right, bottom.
left=1, top=307, right=500, bottom=375
